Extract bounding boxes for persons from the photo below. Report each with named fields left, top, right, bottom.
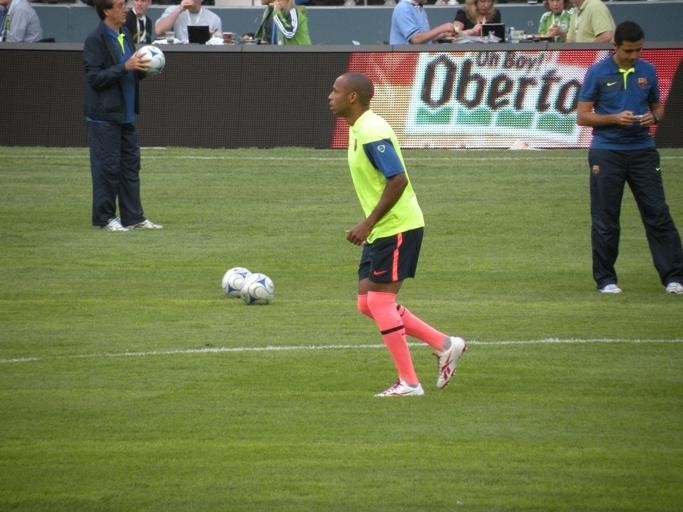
left=122, top=0, right=153, bottom=44
left=271, top=1, right=313, bottom=46
left=154, top=0, right=223, bottom=46
left=453, top=0, right=504, bottom=43
left=536, top=0, right=577, bottom=45
left=552, top=0, right=619, bottom=44
left=0, top=0, right=43, bottom=45
left=80, top=0, right=164, bottom=232
left=326, top=71, right=468, bottom=397
left=575, top=18, right=682, bottom=293
left=388, top=0, right=461, bottom=47
left=237, top=0, right=275, bottom=44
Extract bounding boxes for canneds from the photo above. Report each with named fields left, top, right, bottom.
left=505, top=26, right=514, bottom=42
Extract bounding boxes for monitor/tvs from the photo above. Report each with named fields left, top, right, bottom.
left=187, top=25, right=209, bottom=43
left=482, top=23, right=505, bottom=42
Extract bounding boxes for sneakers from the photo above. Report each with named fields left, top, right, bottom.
left=374, top=377, right=424, bottom=396
left=103, top=217, right=128, bottom=232
left=125, top=219, right=162, bottom=229
left=433, top=336, right=468, bottom=390
left=666, top=282, right=682, bottom=295
left=598, top=284, right=622, bottom=294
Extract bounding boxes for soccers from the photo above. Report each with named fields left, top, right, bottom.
left=137, top=46, right=165, bottom=76
left=241, top=274, right=274, bottom=305
left=222, top=267, right=252, bottom=298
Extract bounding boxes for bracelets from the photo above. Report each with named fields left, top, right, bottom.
left=649, top=110, right=661, bottom=124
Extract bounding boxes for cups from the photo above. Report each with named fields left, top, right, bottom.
left=165, top=31, right=176, bottom=45
left=223, top=31, right=233, bottom=47
